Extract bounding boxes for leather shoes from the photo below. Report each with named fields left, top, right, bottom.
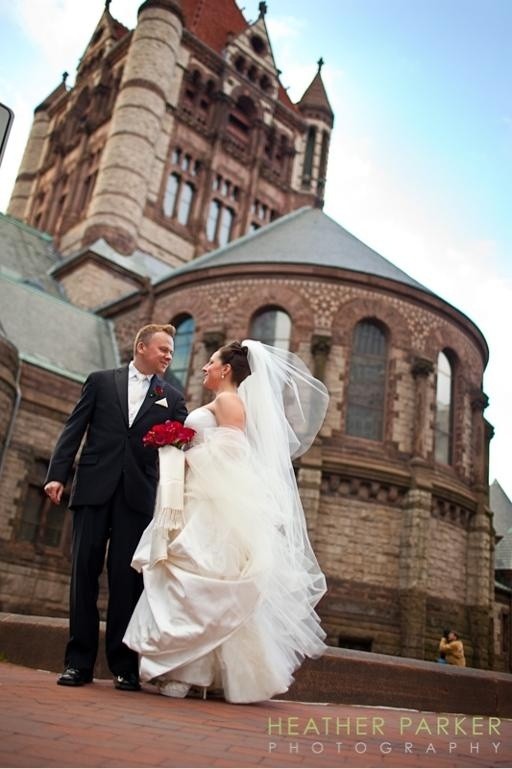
left=57, top=668, right=93, bottom=686
left=114, top=676, right=138, bottom=691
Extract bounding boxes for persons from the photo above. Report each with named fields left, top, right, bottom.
left=41, top=325, right=187, bottom=690
left=438, top=628, right=466, bottom=668
left=119, top=337, right=329, bottom=704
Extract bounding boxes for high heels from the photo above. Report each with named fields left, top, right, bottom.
left=156, top=677, right=207, bottom=699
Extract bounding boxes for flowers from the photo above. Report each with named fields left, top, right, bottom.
left=142, top=419, right=197, bottom=450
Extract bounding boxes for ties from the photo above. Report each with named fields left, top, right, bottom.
left=129, top=374, right=147, bottom=427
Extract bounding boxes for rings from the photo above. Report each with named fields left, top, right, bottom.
left=48, top=493, right=51, bottom=496
